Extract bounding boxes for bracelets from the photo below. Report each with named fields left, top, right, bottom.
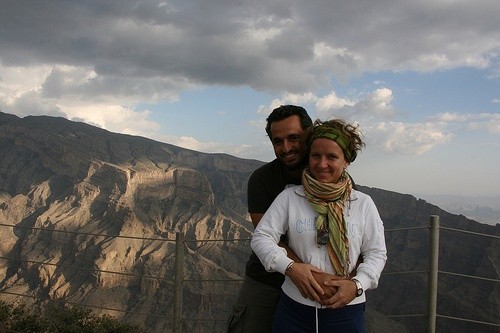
left=284, top=262, right=293, bottom=275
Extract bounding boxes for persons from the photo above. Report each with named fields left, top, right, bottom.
left=249, top=119, right=388, bottom=333
left=226, top=105, right=364, bottom=333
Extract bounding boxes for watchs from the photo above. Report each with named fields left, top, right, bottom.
left=353, top=278, right=363, bottom=297
